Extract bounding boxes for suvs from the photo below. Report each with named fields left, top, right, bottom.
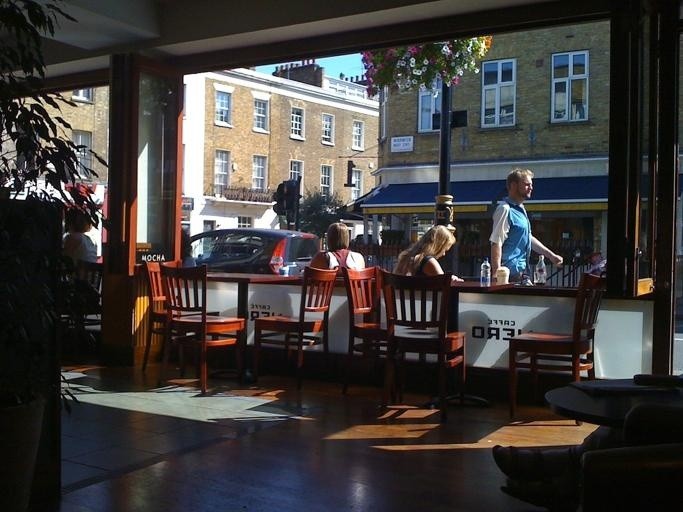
left=185, top=228, right=320, bottom=276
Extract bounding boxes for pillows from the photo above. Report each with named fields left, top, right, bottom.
left=623, top=405, right=683, bottom=445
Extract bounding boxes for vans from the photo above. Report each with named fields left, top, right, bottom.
left=353, top=234, right=383, bottom=252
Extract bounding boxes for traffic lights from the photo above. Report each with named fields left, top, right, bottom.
left=277, top=182, right=297, bottom=209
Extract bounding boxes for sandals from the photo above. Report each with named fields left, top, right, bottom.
left=491, top=442, right=576, bottom=480
left=499, top=483, right=581, bottom=510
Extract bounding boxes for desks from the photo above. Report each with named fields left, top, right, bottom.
left=544, top=374, right=683, bottom=428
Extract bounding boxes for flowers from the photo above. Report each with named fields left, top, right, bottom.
left=360, top=34, right=493, bottom=100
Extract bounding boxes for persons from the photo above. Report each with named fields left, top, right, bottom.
left=62, top=207, right=97, bottom=286
left=489, top=167, right=563, bottom=281
left=587, top=251, right=607, bottom=276
left=392, top=224, right=464, bottom=283
left=309, top=222, right=365, bottom=276
left=180, top=227, right=196, bottom=267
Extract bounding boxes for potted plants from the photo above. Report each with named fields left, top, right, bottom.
left=0, top=0, right=113, bottom=512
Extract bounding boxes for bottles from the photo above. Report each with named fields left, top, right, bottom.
left=535, top=255, right=546, bottom=280
left=480, top=257, right=491, bottom=288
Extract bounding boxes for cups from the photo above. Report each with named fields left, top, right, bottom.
left=495, top=266, right=510, bottom=285
left=533, top=272, right=546, bottom=287
left=279, top=267, right=289, bottom=276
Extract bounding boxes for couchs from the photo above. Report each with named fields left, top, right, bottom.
left=492, top=403, right=683, bottom=512
